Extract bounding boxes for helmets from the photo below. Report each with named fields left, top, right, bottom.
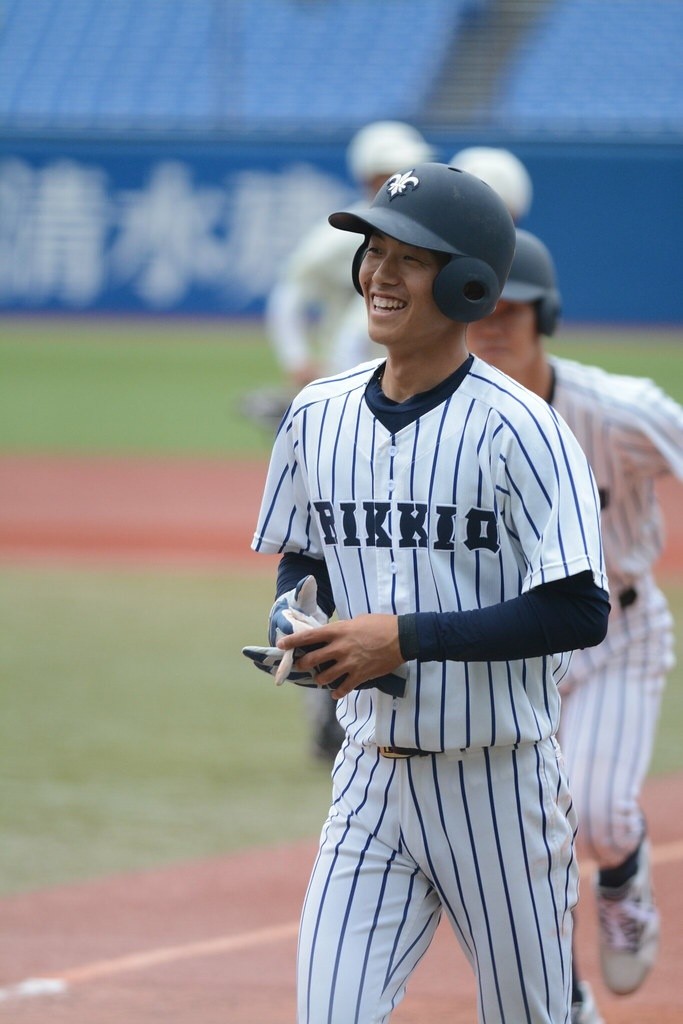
left=501, top=230, right=561, bottom=338
left=347, top=122, right=438, bottom=180
left=450, top=145, right=532, bottom=217
left=328, top=165, right=517, bottom=323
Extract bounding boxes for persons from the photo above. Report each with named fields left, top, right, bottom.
left=465, top=226, right=682, bottom=1021
left=266, top=167, right=608, bottom=1021
left=266, top=119, right=533, bottom=767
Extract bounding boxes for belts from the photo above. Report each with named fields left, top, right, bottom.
left=376, top=744, right=439, bottom=760
left=619, top=587, right=637, bottom=607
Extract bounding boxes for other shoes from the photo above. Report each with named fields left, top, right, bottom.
left=318, top=719, right=346, bottom=759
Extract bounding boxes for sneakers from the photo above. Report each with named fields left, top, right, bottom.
left=593, top=840, right=659, bottom=994
left=571, top=981, right=605, bottom=1024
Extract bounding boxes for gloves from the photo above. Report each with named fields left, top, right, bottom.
left=243, top=575, right=409, bottom=701
